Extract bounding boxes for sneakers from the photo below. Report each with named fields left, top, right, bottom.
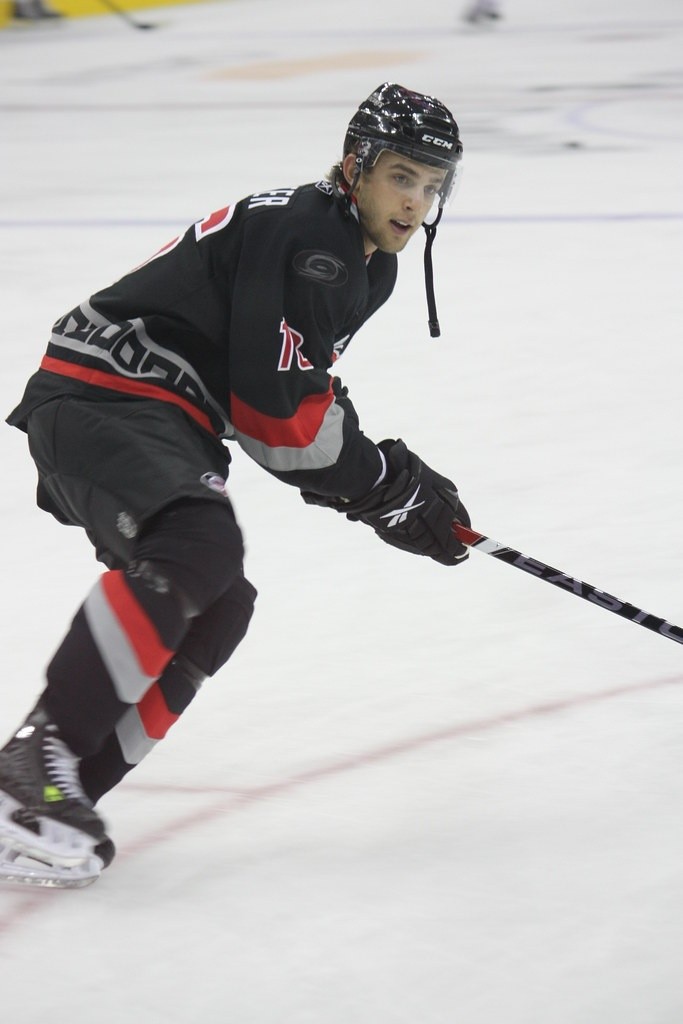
left=0, top=699, right=108, bottom=866
left=0, top=838, right=116, bottom=889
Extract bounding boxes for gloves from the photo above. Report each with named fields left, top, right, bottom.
left=300, top=438, right=471, bottom=566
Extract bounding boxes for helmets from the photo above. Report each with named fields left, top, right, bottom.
left=332, top=81, right=463, bottom=231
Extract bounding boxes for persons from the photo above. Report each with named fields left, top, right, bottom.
left=0, top=84, right=475, bottom=889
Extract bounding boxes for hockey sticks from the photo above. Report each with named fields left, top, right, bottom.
left=452, top=522, right=683, bottom=644
left=103, top=0, right=160, bottom=30
